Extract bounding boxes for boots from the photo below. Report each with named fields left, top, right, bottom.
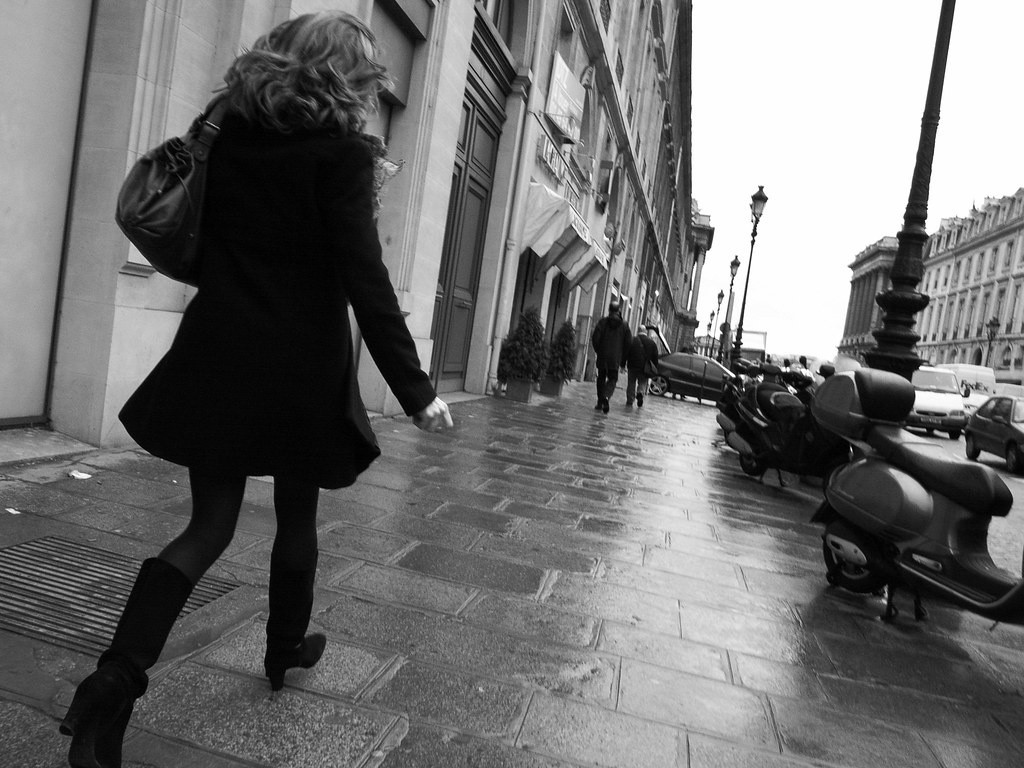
left=264, top=549, right=326, bottom=691
left=59, top=557, right=192, bottom=768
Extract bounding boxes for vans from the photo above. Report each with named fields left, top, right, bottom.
left=937, top=363, right=996, bottom=421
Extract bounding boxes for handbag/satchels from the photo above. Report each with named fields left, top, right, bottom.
left=114, top=97, right=230, bottom=287
left=644, top=359, right=657, bottom=378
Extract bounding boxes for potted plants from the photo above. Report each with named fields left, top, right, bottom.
left=497, top=303, right=549, bottom=403
left=541, top=316, right=579, bottom=397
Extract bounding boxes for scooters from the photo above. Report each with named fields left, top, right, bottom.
left=726, top=357, right=866, bottom=485
left=808, top=360, right=1024, bottom=635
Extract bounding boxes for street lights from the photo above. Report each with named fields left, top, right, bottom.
left=983, top=317, right=1002, bottom=371
left=728, top=184, right=770, bottom=371
left=716, top=255, right=741, bottom=366
left=704, top=290, right=726, bottom=358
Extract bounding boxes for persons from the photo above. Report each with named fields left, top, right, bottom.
left=779, top=356, right=813, bottom=380
left=766, top=354, right=771, bottom=364
left=58, top=10, right=456, bottom=768
left=593, top=301, right=632, bottom=413
left=672, top=341, right=692, bottom=401
left=620, top=324, right=659, bottom=408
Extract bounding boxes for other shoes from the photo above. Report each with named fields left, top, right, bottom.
left=637, top=393, right=644, bottom=407
left=595, top=404, right=602, bottom=410
left=601, top=396, right=609, bottom=414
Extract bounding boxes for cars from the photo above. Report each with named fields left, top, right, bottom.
left=908, top=366, right=968, bottom=438
left=645, top=325, right=744, bottom=406
left=964, top=394, right=1024, bottom=470
